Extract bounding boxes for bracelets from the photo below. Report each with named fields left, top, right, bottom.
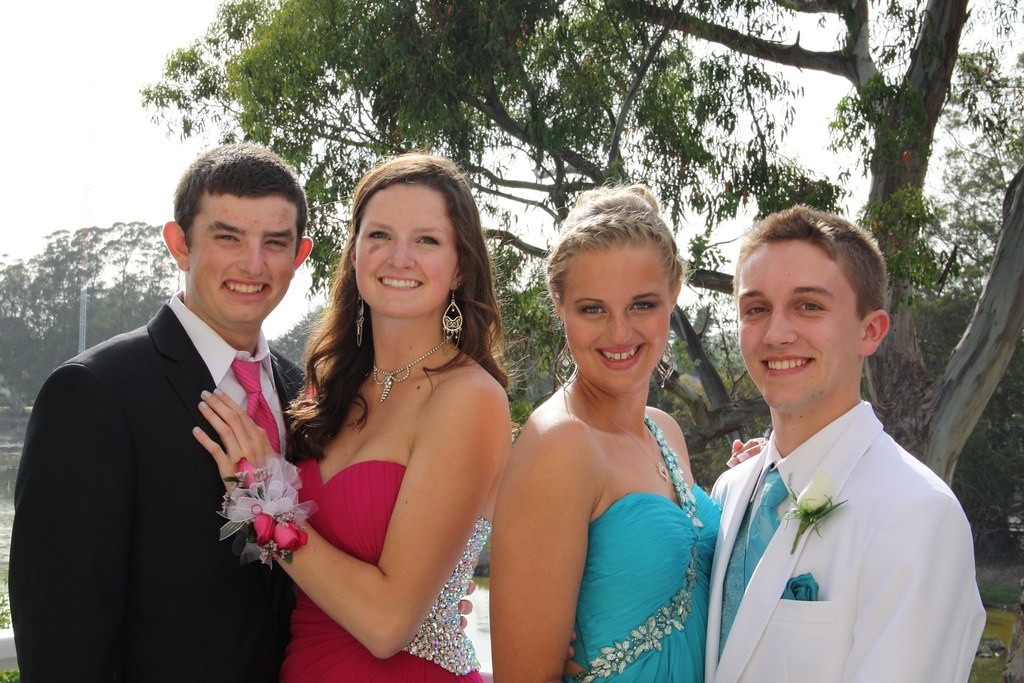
left=213, top=450, right=315, bottom=568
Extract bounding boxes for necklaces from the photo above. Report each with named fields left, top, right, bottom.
left=366, top=336, right=452, bottom=404
left=576, top=372, right=670, bottom=483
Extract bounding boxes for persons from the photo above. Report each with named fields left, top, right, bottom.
left=8, top=138, right=478, bottom=681
left=483, top=185, right=769, bottom=683
left=702, top=202, right=985, bottom=683
left=186, top=145, right=519, bottom=683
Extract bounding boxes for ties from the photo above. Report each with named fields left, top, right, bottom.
left=231, top=357, right=281, bottom=459
left=745, top=467, right=790, bottom=590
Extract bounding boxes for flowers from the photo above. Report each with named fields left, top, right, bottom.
left=783, top=469, right=848, bottom=554
left=217, top=457, right=314, bottom=570
